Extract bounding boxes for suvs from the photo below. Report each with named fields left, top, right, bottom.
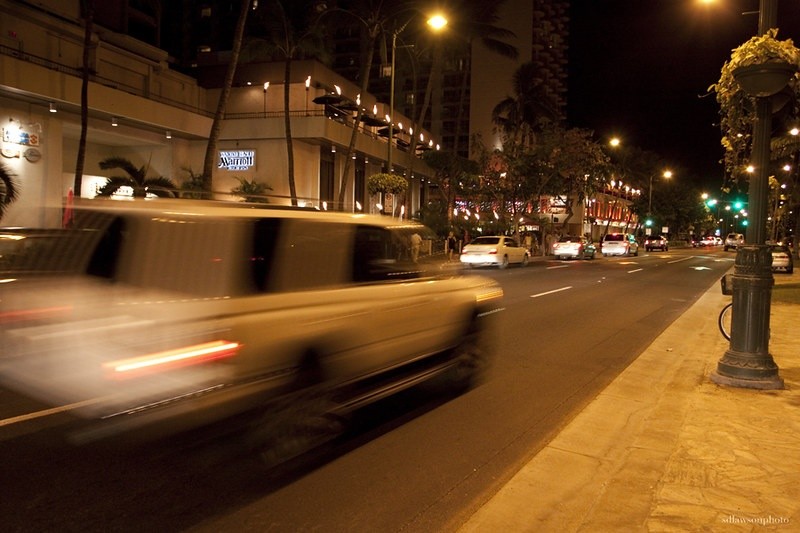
left=601, top=233, right=640, bottom=258
left=723, top=233, right=745, bottom=251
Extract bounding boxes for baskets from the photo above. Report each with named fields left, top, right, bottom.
left=721, top=274, right=733, bottom=295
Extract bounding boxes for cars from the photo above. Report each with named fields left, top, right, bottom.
left=458, top=235, right=531, bottom=269
left=552, top=236, right=598, bottom=260
left=0, top=201, right=504, bottom=454
left=698, top=237, right=724, bottom=246
left=645, top=235, right=669, bottom=252
left=771, top=246, right=794, bottom=273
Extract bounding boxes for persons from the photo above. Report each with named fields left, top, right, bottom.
left=446, top=230, right=457, bottom=260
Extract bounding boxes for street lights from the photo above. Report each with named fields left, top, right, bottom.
left=386, top=9, right=449, bottom=210
left=648, top=171, right=673, bottom=216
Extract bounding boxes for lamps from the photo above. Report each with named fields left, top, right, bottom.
left=166, top=130, right=172, bottom=138
left=263, top=82, right=269, bottom=93
left=112, top=117, right=119, bottom=126
left=306, top=76, right=312, bottom=91
left=50, top=102, right=57, bottom=112
left=391, top=166, right=431, bottom=183
left=352, top=153, right=356, bottom=159
left=332, top=146, right=336, bottom=152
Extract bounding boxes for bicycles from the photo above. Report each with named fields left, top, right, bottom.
left=718, top=277, right=733, bottom=342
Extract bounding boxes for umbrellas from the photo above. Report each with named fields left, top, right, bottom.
left=312, top=93, right=400, bottom=138
